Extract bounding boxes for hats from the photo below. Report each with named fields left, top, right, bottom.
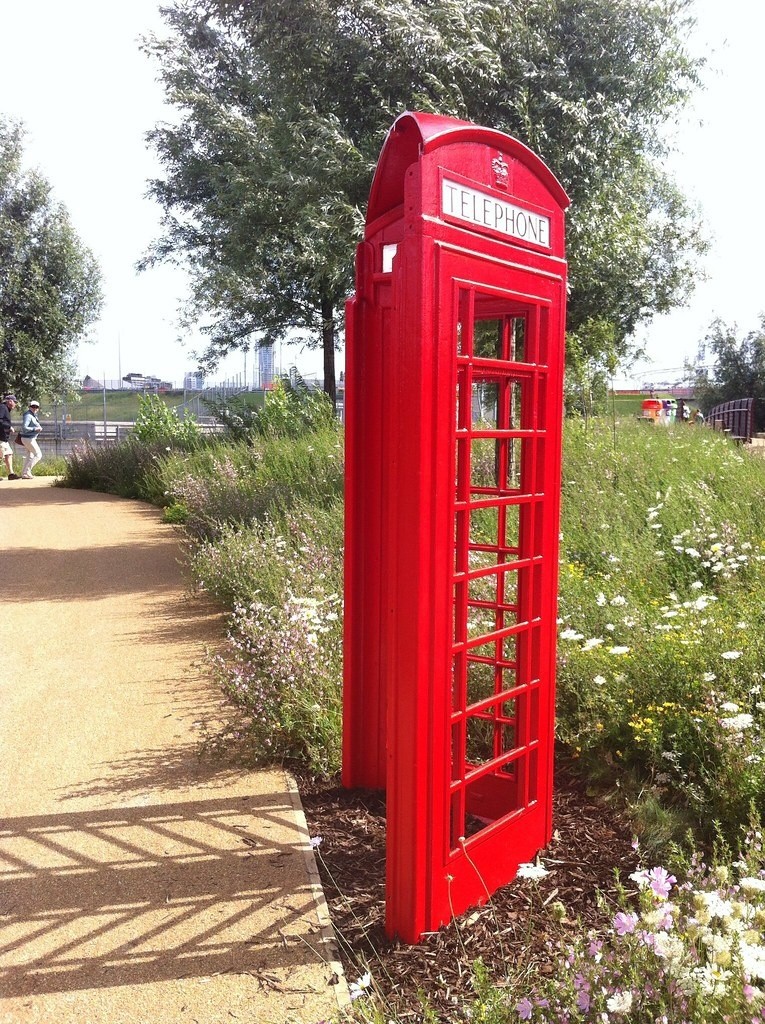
left=2, top=395, right=16, bottom=403
left=29, top=401, right=39, bottom=406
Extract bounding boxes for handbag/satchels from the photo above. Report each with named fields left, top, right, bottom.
left=14, top=433, right=24, bottom=446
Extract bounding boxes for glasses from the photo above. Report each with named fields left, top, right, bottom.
left=30, top=405, right=39, bottom=408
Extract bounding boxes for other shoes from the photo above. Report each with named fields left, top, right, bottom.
left=22, top=474, right=35, bottom=479
left=0, top=477, right=3, bottom=481
left=8, top=474, right=19, bottom=480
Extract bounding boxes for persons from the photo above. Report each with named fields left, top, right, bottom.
left=0, top=395, right=23, bottom=481
left=662, top=399, right=704, bottom=422
left=20, top=400, right=42, bottom=479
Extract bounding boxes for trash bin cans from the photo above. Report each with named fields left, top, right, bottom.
left=641, top=398, right=678, bottom=425
